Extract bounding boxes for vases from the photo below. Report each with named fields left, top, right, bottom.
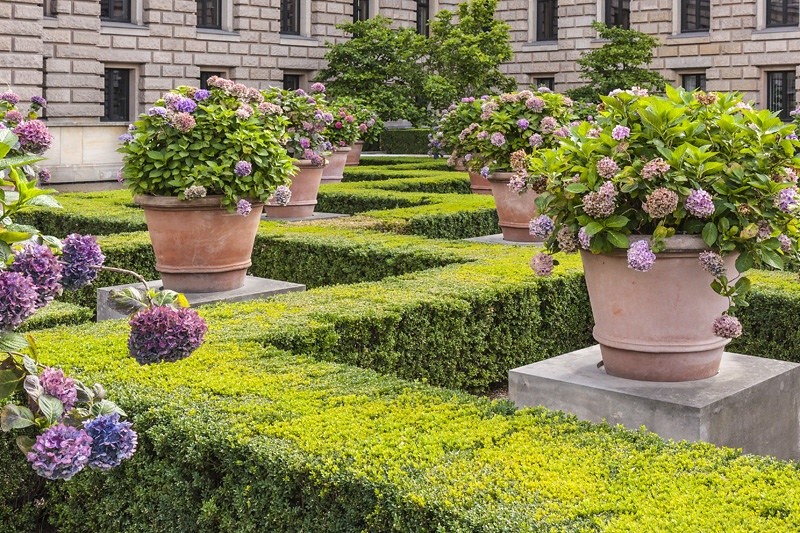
left=265, top=159, right=329, bottom=217
left=489, top=173, right=556, bottom=241
left=135, top=195, right=266, bottom=292
left=305, top=147, right=351, bottom=182
left=469, top=171, right=492, bottom=194
left=577, top=235, right=744, bottom=381
left=345, top=141, right=364, bottom=166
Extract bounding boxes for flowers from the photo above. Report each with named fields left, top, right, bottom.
left=0, top=75, right=800, bottom=481
